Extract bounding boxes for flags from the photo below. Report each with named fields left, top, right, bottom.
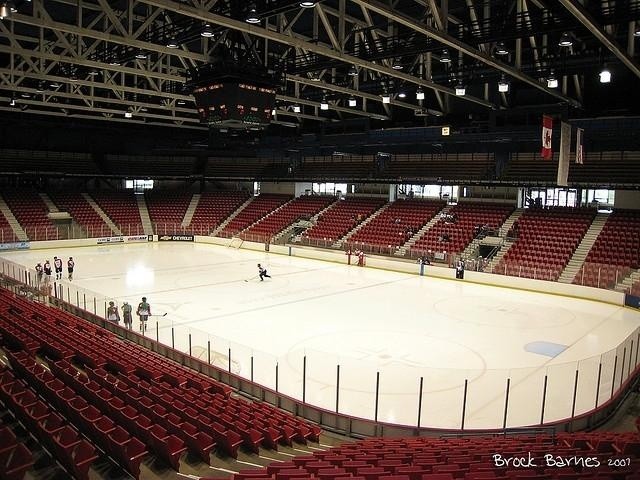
left=575, top=128, right=584, bottom=164
left=541, top=116, right=552, bottom=160
left=557, top=122, right=572, bottom=187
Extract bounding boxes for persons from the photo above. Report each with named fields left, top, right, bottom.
left=459, top=260, right=465, bottom=270
left=257, top=263, right=271, bottom=281
left=417, top=255, right=431, bottom=275
left=350, top=209, right=518, bottom=242
left=345, top=248, right=364, bottom=266
left=107, top=297, right=152, bottom=331
left=36, top=256, right=75, bottom=280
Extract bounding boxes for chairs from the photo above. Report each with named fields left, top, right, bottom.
left=1, top=186, right=387, bottom=250
left=350, top=196, right=638, bottom=289
left=199, top=435, right=640, bottom=480
left=0, top=331, right=321, bottom=479
